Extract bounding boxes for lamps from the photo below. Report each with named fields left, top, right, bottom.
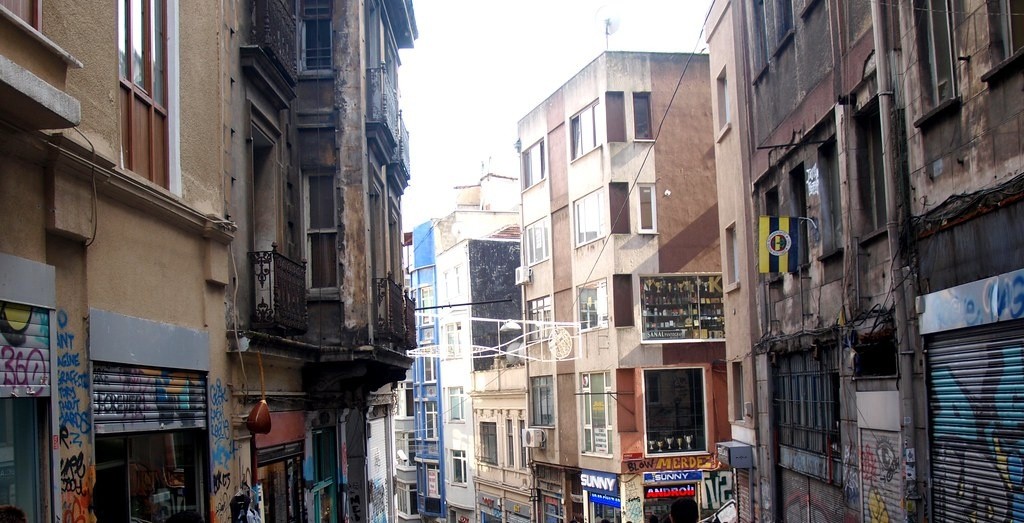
left=528, top=487, right=540, bottom=502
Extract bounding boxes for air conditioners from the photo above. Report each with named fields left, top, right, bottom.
left=515, top=266, right=531, bottom=286
left=522, top=429, right=541, bottom=447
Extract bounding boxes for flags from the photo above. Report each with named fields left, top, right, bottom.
left=759, top=215, right=800, bottom=273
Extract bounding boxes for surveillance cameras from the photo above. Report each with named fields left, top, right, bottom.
left=397, top=450, right=408, bottom=462
